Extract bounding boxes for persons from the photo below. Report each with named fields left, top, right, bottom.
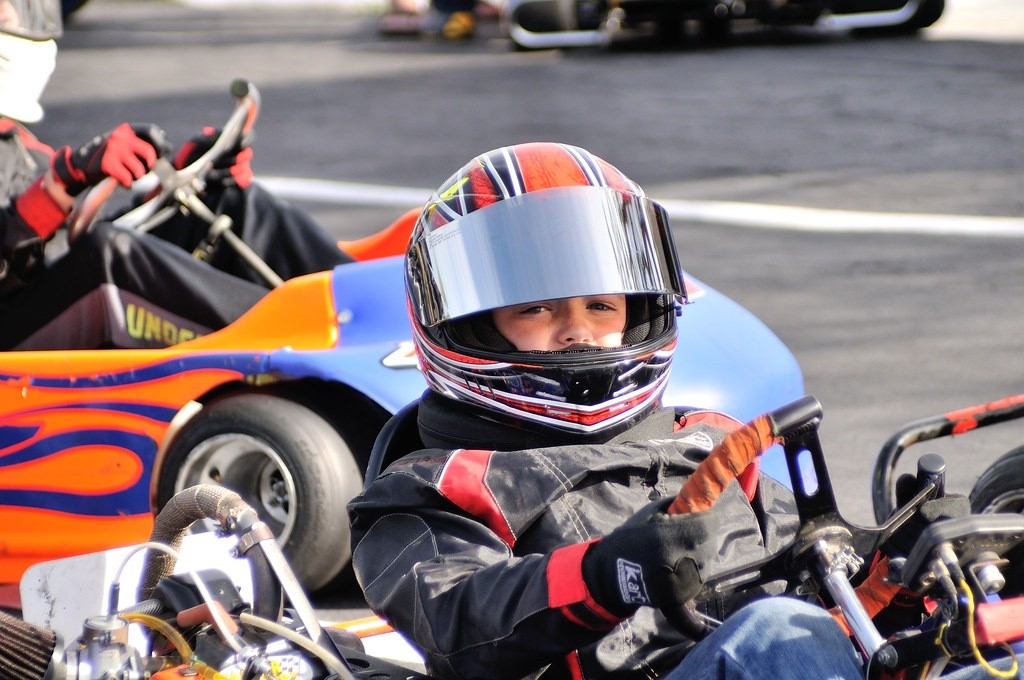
left=2, top=0, right=358, bottom=353
left=347, top=142, right=1023, bottom=680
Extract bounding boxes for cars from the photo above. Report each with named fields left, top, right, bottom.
left=3, top=193, right=1024, bottom=680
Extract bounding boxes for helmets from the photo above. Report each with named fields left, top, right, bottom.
left=402, top=144, right=686, bottom=439
left=0, top=0, right=63, bottom=124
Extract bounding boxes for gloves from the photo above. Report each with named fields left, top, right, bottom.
left=51, top=120, right=166, bottom=189
left=174, top=125, right=255, bottom=194
left=887, top=474, right=971, bottom=583
left=589, top=496, right=717, bottom=612
